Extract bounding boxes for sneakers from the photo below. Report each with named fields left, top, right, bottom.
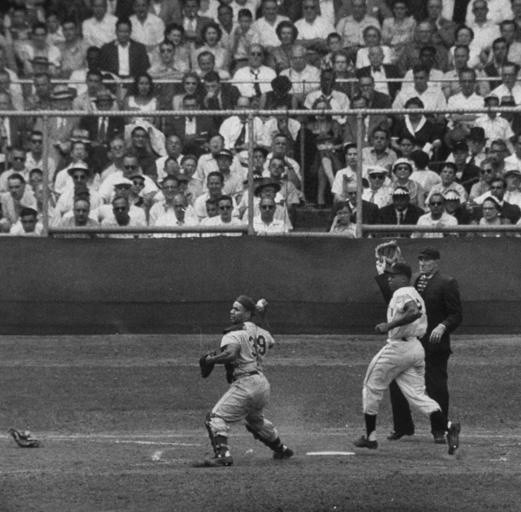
left=444, top=421, right=461, bottom=455
left=432, top=430, right=446, bottom=444
left=387, top=424, right=414, bottom=440
left=211, top=455, right=233, bottom=465
left=273, top=447, right=292, bottom=459
left=352, top=435, right=378, bottom=449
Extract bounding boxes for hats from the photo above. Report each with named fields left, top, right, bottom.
left=418, top=246, right=440, bottom=260
left=254, top=178, right=281, bottom=196
left=28, top=56, right=54, bottom=65
left=113, top=177, right=134, bottom=187
left=384, top=261, right=412, bottom=276
left=69, top=129, right=93, bottom=144
left=367, top=127, right=490, bottom=200
left=91, top=93, right=116, bottom=102
left=50, top=85, right=74, bottom=99
left=237, top=294, right=256, bottom=315
left=213, top=148, right=233, bottom=159
left=67, top=159, right=90, bottom=176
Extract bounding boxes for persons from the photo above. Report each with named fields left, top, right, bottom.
left=0, top=0, right=520, bottom=237
left=199, top=294, right=295, bottom=467
left=374, top=240, right=463, bottom=443
left=351, top=262, right=461, bottom=456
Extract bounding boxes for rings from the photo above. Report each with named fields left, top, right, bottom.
left=435, top=334, right=438, bottom=338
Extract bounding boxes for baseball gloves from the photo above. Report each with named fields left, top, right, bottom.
left=201, top=351, right=213, bottom=378
left=9, top=428, right=39, bottom=448
left=375, top=241, right=400, bottom=266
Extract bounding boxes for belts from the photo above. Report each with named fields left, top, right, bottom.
left=232, top=371, right=259, bottom=381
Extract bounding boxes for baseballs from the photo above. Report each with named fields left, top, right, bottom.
left=257, top=299, right=264, bottom=307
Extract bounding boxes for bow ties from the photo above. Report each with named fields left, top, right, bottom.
left=373, top=67, right=381, bottom=73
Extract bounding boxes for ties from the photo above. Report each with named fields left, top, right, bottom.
left=252, top=69, right=262, bottom=97
left=99, top=116, right=106, bottom=142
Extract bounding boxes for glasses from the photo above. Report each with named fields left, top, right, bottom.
left=261, top=205, right=274, bottom=210
left=160, top=48, right=172, bottom=53
left=124, top=165, right=136, bottom=170
left=220, top=206, right=232, bottom=210
left=251, top=53, right=261, bottom=57
left=115, top=206, right=127, bottom=212
